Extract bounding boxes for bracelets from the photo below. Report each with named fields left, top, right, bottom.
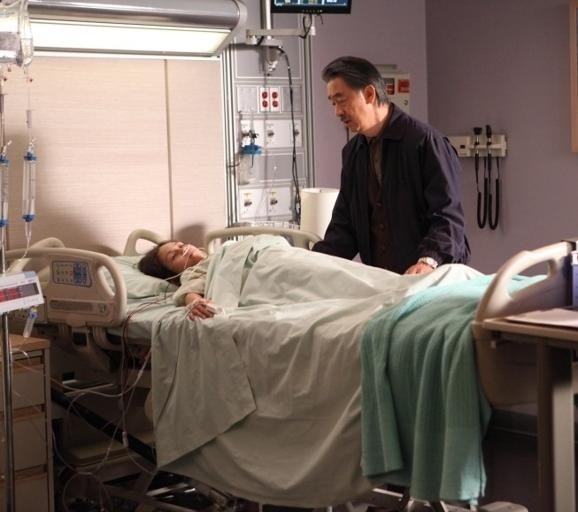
left=417, top=256, right=440, bottom=270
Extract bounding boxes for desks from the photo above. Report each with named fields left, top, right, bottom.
left=477, top=309, right=578, bottom=512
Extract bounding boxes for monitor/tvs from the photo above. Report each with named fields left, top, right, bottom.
left=271, top=0, right=351, bottom=14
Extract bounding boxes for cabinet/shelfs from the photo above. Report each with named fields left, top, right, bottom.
left=0, top=332, right=58, bottom=510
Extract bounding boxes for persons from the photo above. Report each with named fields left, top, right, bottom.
left=138, top=238, right=224, bottom=323
left=313, top=55, right=471, bottom=275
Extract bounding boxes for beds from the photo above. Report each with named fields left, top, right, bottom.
left=1, top=226, right=577, bottom=510
